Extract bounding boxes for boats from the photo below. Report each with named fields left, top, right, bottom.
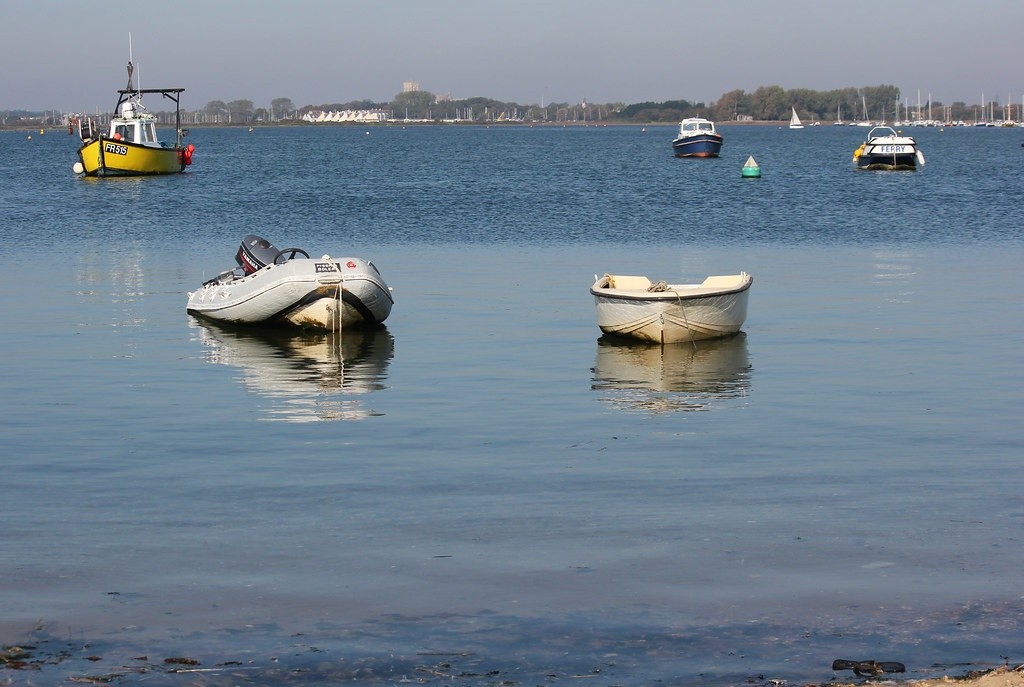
left=192, top=318, right=394, bottom=418
left=77, top=31, right=188, bottom=177
left=854, top=126, right=917, bottom=171
left=673, top=116, right=723, bottom=158
left=592, top=270, right=753, bottom=345
left=592, top=330, right=751, bottom=413
left=186, top=235, right=394, bottom=330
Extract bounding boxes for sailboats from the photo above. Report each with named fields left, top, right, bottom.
left=849, top=102, right=858, bottom=126
left=834, top=104, right=844, bottom=125
left=857, top=96, right=873, bottom=127
left=789, top=106, right=805, bottom=128
left=875, top=88, right=1024, bottom=127
